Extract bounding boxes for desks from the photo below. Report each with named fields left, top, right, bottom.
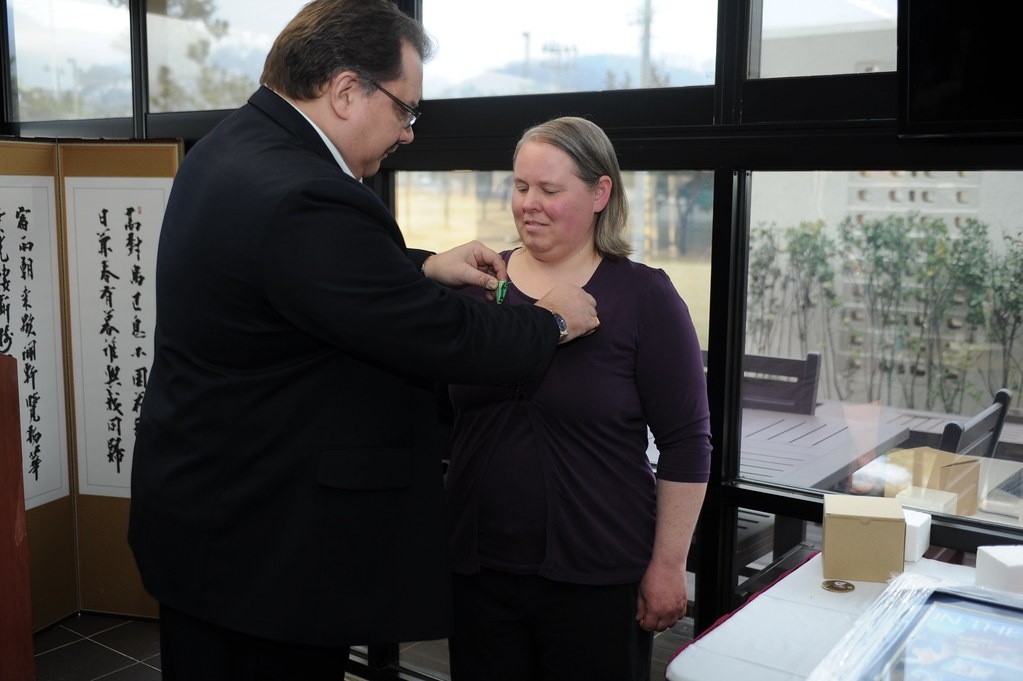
left=968, top=454, right=1023, bottom=529
left=644, top=408, right=910, bottom=620
left=665, top=552, right=975, bottom=681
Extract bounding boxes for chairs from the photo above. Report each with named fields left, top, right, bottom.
left=681, top=350, right=822, bottom=580
left=736, top=388, right=1014, bottom=600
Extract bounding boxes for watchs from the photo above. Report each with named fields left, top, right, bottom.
left=547, top=309, right=568, bottom=343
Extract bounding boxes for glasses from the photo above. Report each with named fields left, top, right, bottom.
left=349, top=67, right=421, bottom=128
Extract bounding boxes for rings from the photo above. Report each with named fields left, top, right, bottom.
left=594, top=317, right=600, bottom=327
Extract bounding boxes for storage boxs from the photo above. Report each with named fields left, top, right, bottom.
left=822, top=494, right=906, bottom=583
left=895, top=486, right=958, bottom=516
left=883, top=446, right=980, bottom=516
left=902, top=509, right=931, bottom=563
left=975, top=545, right=1023, bottom=595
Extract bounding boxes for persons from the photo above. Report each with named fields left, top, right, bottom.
left=127, top=0, right=600, bottom=681
left=435, top=115, right=712, bottom=680
